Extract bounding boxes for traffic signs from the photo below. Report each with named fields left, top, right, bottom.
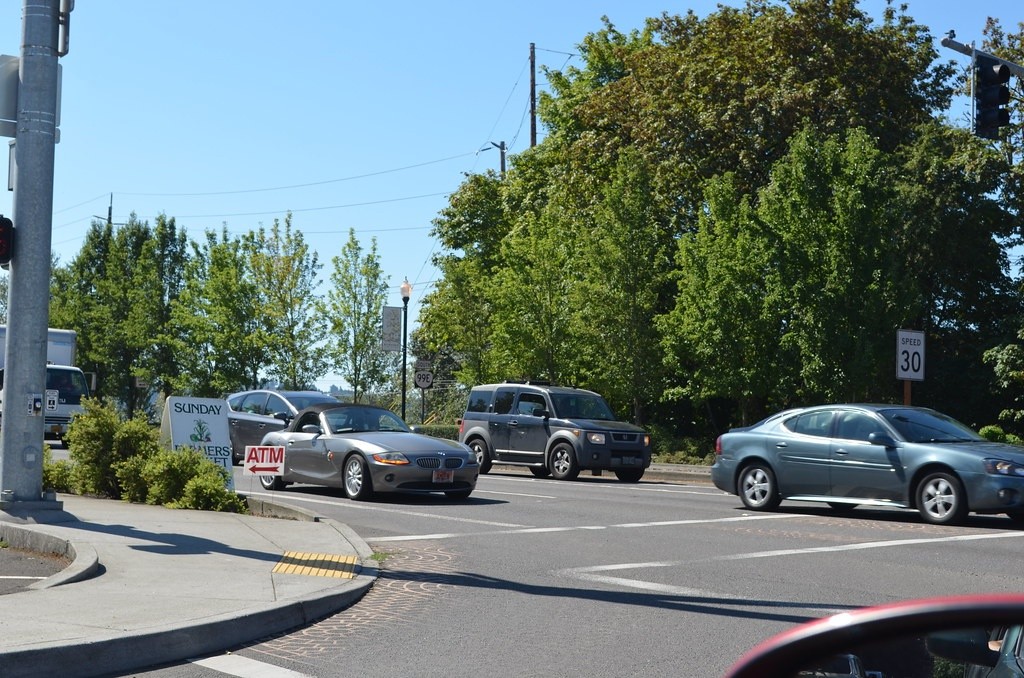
left=242, top=446, right=285, bottom=476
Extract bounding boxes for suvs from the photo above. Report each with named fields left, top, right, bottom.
left=457, top=379, right=653, bottom=482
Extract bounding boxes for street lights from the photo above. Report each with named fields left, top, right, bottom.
left=401, top=276, right=410, bottom=424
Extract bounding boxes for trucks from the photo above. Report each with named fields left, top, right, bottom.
left=0, top=325, right=97, bottom=449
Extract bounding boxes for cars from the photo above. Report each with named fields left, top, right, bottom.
left=711, top=403, right=1024, bottom=525
left=226, top=390, right=352, bottom=465
left=259, top=404, right=481, bottom=500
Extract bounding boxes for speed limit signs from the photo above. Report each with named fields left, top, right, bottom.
left=896, top=329, right=926, bottom=382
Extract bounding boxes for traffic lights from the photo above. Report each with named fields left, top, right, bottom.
left=974, top=56, right=1009, bottom=139
